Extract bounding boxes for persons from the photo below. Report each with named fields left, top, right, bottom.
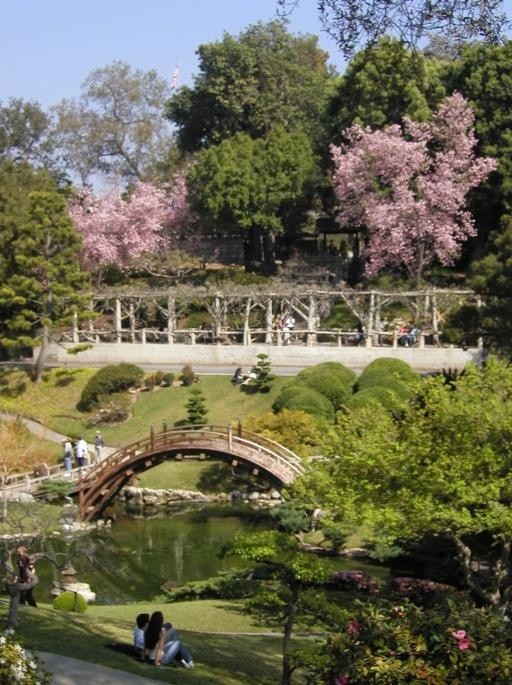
left=18, top=546, right=38, bottom=607
left=141, top=612, right=194, bottom=669
left=272, top=311, right=295, bottom=346
left=62, top=430, right=104, bottom=470
left=134, top=614, right=177, bottom=657
left=348, top=318, right=420, bottom=347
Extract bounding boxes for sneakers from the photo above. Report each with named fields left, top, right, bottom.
left=181, top=659, right=194, bottom=671
